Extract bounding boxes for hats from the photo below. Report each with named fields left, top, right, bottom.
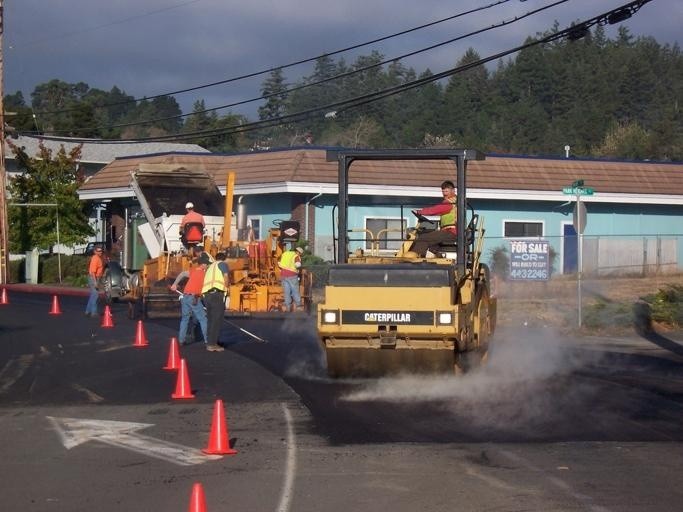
left=194, top=246, right=202, bottom=252
left=296, top=247, right=303, bottom=253
left=198, top=257, right=212, bottom=264
left=185, top=202, right=194, bottom=209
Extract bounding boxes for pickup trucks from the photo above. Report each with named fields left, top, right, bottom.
left=72, top=242, right=105, bottom=257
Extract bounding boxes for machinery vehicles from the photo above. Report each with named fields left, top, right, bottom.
left=101, top=170, right=312, bottom=319
left=316, top=149, right=497, bottom=377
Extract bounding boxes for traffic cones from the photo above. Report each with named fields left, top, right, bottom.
left=171, top=357, right=196, bottom=399
left=0, top=287, right=9, bottom=304
left=189, top=482, right=209, bottom=512
left=163, top=337, right=180, bottom=370
left=101, top=305, right=114, bottom=328
left=48, top=295, right=62, bottom=313
left=132, top=320, right=150, bottom=346
left=202, top=399, right=238, bottom=454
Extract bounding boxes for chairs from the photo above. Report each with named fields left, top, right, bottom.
left=181, top=223, right=204, bottom=247
left=438, top=213, right=478, bottom=247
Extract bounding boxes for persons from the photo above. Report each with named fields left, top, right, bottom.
left=84, top=246, right=105, bottom=318
left=278, top=246, right=303, bottom=312
left=171, top=259, right=212, bottom=346
left=201, top=253, right=230, bottom=352
left=408, top=181, right=467, bottom=258
left=192, top=246, right=215, bottom=264
left=179, top=201, right=205, bottom=248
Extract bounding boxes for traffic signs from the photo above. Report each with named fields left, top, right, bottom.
left=572, top=179, right=584, bottom=187
left=563, top=185, right=594, bottom=196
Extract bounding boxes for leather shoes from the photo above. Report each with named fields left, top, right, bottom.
left=206, top=344, right=224, bottom=352
